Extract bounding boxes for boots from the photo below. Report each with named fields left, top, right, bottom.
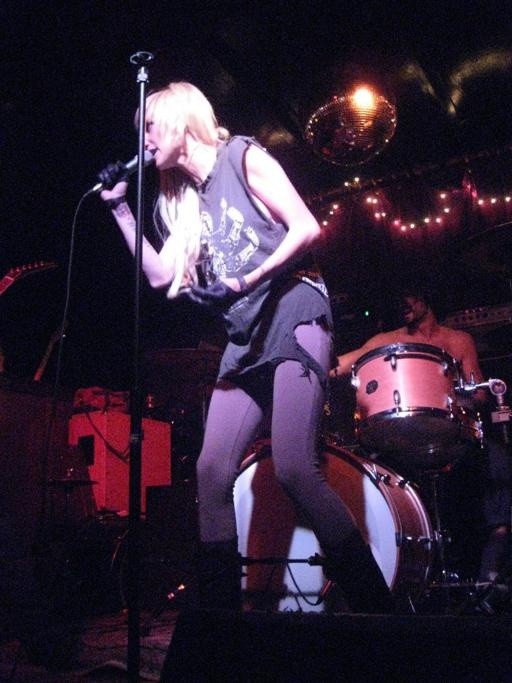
left=187, top=538, right=242, bottom=611
left=321, top=548, right=394, bottom=608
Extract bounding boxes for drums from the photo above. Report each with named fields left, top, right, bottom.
left=230, top=442, right=436, bottom=615
left=352, top=343, right=477, bottom=469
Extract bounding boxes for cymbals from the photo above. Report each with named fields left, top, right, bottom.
left=159, top=349, right=224, bottom=362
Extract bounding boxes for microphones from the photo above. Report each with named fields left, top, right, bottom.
left=82, top=150, right=155, bottom=202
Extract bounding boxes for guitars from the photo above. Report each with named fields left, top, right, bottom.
left=0, top=260, right=59, bottom=293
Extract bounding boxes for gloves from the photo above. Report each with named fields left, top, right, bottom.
left=97, top=159, right=136, bottom=208
left=185, top=277, right=246, bottom=311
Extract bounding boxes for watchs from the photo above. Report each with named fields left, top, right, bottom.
left=226, top=272, right=246, bottom=298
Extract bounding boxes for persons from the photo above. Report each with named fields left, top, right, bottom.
left=93, top=84, right=388, bottom=614
left=321, top=265, right=482, bottom=441
